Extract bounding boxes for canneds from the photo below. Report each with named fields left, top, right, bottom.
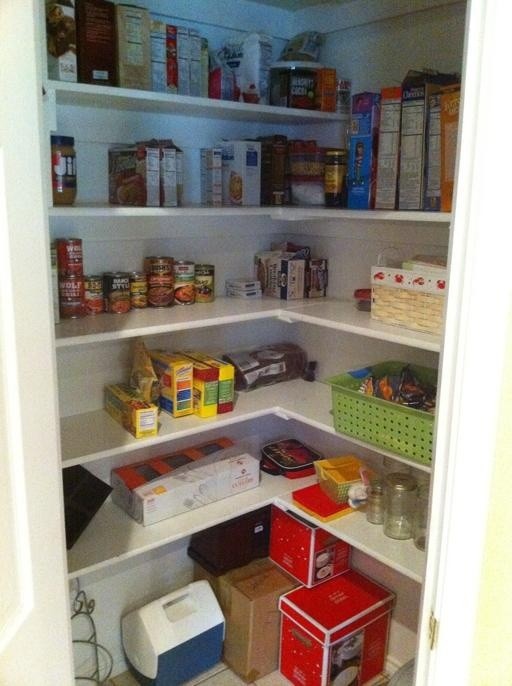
left=55, top=238, right=84, bottom=277
left=84, top=275, right=105, bottom=315
left=103, top=271, right=131, bottom=314
left=129, top=271, right=148, bottom=309
left=173, top=260, right=196, bottom=306
left=194, top=264, right=215, bottom=304
left=143, top=255, right=175, bottom=309
left=58, top=277, right=84, bottom=319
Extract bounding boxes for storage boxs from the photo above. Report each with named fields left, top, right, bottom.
left=279, top=572, right=396, bottom=686
left=219, top=559, right=299, bottom=684
left=267, top=505, right=354, bottom=586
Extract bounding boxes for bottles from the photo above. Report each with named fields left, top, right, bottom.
left=381, top=472, right=419, bottom=541
left=50, top=136, right=77, bottom=204
left=323, top=149, right=347, bottom=207
left=365, top=479, right=385, bottom=525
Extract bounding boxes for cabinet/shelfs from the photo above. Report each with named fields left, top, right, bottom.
left=2, top=0, right=507, bottom=686
